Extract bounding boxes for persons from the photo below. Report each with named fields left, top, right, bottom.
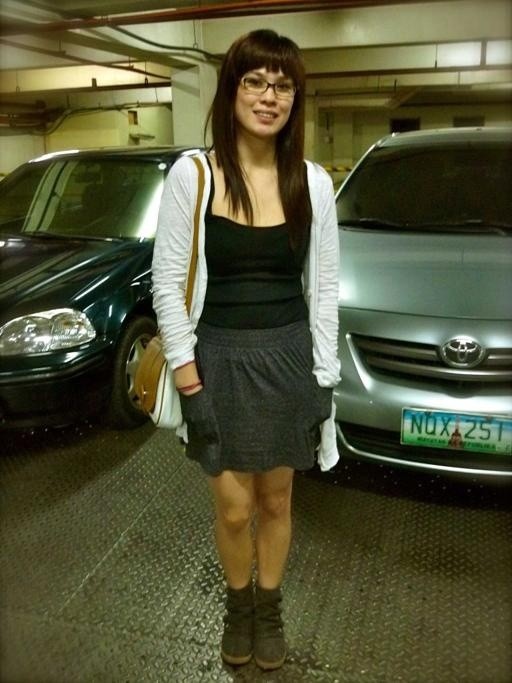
left=149, top=26, right=341, bottom=671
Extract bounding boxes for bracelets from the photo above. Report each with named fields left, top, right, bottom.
left=174, top=379, right=203, bottom=393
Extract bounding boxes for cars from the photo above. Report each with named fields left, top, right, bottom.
left=1, top=144, right=199, bottom=437
left=313, top=125, right=512, bottom=493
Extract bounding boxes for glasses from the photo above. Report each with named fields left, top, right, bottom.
left=241, top=77, right=298, bottom=98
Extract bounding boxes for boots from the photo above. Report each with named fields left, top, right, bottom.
left=254, top=581, right=287, bottom=670
left=220, top=579, right=254, bottom=664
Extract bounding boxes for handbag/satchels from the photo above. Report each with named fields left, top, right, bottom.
left=134, top=334, right=184, bottom=430
